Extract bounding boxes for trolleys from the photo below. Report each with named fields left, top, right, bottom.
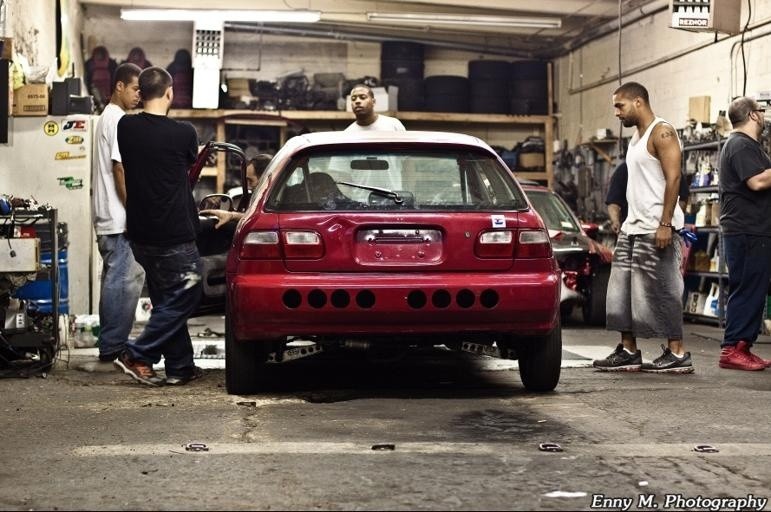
left=0, top=204, right=61, bottom=375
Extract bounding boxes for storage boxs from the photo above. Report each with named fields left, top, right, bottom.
left=13, top=84, right=48, bottom=117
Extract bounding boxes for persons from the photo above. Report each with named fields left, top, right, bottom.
left=117, top=66, right=204, bottom=387
left=593, top=82, right=694, bottom=375
left=606, top=161, right=690, bottom=234
left=91, top=63, right=143, bottom=362
left=328, top=84, right=416, bottom=204
left=198, top=154, right=273, bottom=229
left=719, top=96, right=771, bottom=371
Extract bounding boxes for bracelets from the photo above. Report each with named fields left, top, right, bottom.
left=660, top=221, right=671, bottom=227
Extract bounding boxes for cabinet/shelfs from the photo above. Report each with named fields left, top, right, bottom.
left=168, top=108, right=557, bottom=194
left=683, top=138, right=730, bottom=327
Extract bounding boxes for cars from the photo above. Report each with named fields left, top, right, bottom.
left=429, top=177, right=610, bottom=324
left=176, top=129, right=566, bottom=395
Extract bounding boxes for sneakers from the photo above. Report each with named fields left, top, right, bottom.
left=719, top=341, right=771, bottom=371
left=114, top=350, right=168, bottom=387
left=641, top=344, right=694, bottom=374
left=593, top=343, right=642, bottom=371
left=166, top=366, right=204, bottom=385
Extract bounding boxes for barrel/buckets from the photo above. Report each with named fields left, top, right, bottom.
left=686, top=286, right=707, bottom=316
left=0, top=222, right=71, bottom=316
left=708, top=249, right=729, bottom=274
left=15, top=312, right=71, bottom=348
left=702, top=281, right=729, bottom=319
